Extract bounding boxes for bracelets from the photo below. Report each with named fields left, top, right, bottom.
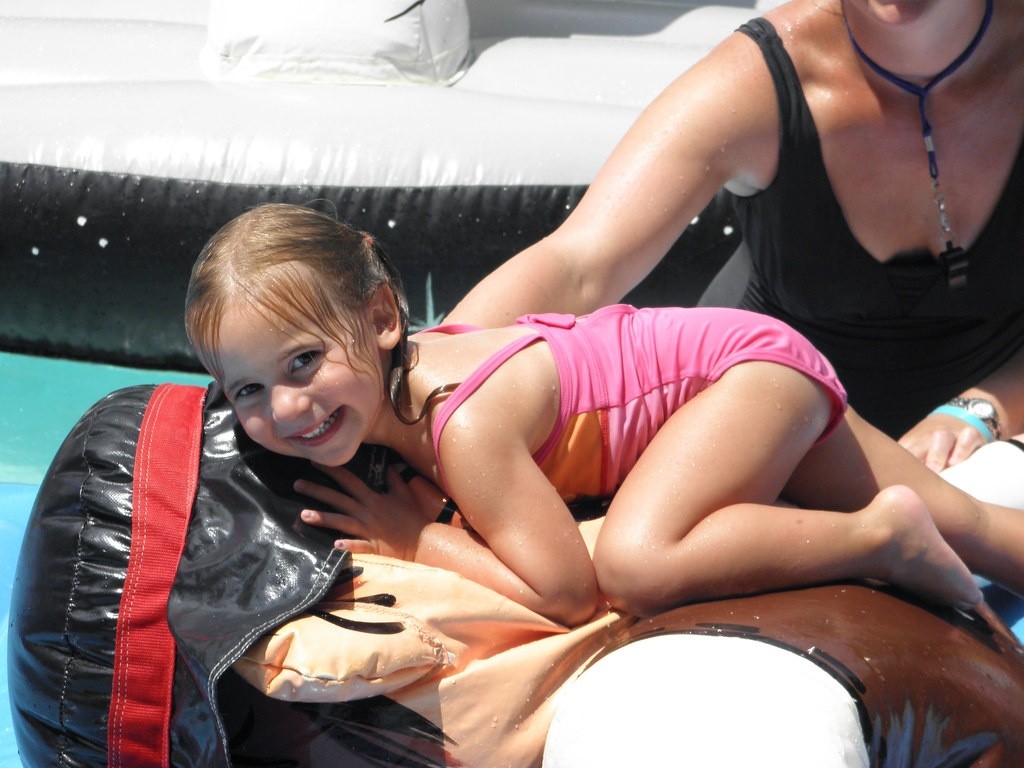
left=929, top=405, right=993, bottom=444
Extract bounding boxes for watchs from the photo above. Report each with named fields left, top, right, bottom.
left=947, top=397, right=1002, bottom=440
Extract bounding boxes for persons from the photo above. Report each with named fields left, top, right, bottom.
left=442, top=1, right=1024, bottom=472
left=186, top=203, right=1024, bottom=624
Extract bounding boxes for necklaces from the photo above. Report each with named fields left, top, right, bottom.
left=840, top=1, right=993, bottom=299
